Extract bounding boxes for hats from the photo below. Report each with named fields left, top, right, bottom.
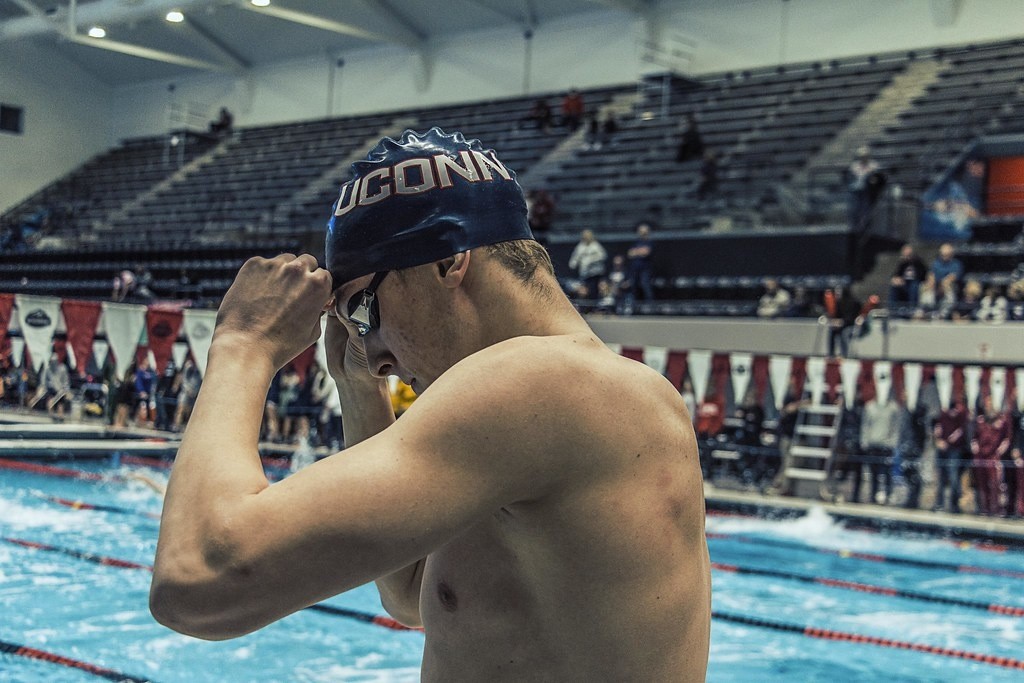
left=326, top=126, right=536, bottom=295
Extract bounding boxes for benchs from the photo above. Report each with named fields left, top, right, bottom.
left=0, top=35, right=1023, bottom=326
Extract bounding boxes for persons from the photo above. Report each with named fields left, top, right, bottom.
left=150, top=126, right=711, bottom=683
left=681, top=375, right=1024, bottom=521
left=758, top=148, right=1024, bottom=321
left=0, top=270, right=158, bottom=425
left=209, top=107, right=232, bottom=132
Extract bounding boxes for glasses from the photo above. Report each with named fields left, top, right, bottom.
left=331, top=270, right=394, bottom=337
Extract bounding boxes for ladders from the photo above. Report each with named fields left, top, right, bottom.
left=780, top=312, right=865, bottom=497
